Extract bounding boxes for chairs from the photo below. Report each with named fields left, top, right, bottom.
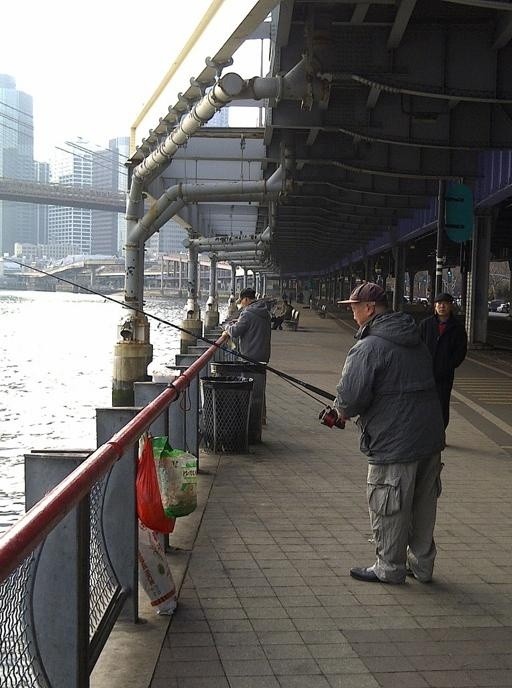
left=283, top=308, right=300, bottom=332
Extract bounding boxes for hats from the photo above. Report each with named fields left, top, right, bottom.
left=338, top=282, right=388, bottom=304
left=435, top=293, right=452, bottom=302
left=236, top=288, right=255, bottom=303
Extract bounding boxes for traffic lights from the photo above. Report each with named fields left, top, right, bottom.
left=447, top=271, right=452, bottom=282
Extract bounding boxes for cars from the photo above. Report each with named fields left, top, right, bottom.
left=488, top=299, right=511, bottom=313
left=404, top=295, right=428, bottom=309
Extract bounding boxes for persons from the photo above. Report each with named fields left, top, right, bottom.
left=333, top=282, right=446, bottom=586
left=418, top=292, right=468, bottom=430
left=225, top=287, right=271, bottom=425
left=271, top=300, right=294, bottom=330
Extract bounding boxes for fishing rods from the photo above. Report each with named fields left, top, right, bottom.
left=0, top=256, right=352, bottom=429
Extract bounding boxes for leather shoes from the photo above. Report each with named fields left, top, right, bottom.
left=351, top=567, right=381, bottom=582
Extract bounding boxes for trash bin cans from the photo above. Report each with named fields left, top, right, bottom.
left=200, top=361, right=267, bottom=455
